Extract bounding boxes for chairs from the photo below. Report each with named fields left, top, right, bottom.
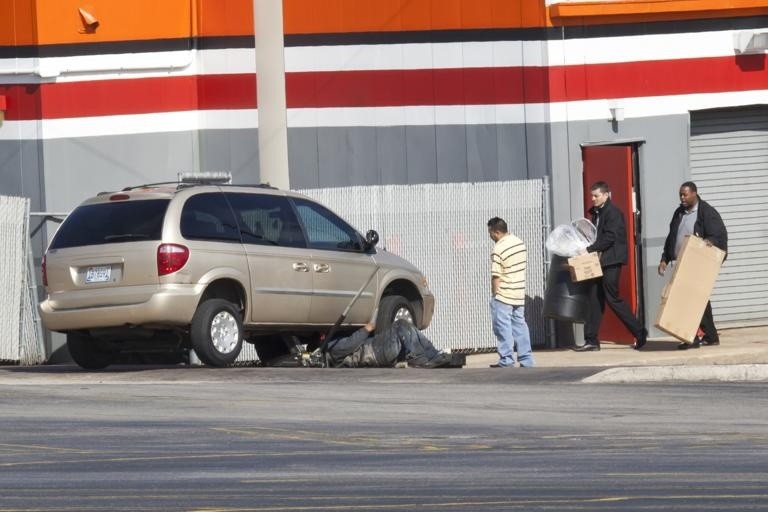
left=277, top=228, right=305, bottom=244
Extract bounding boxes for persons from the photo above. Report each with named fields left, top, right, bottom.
left=655, top=179, right=729, bottom=352
left=305, top=306, right=466, bottom=369
left=484, top=214, right=537, bottom=370
left=566, top=179, right=649, bottom=354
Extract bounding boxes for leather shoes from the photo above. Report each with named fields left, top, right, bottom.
left=677, top=336, right=720, bottom=349
left=575, top=343, right=601, bottom=351
left=633, top=328, right=648, bottom=349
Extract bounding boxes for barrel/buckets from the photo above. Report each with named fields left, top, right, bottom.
left=543, top=255, right=598, bottom=323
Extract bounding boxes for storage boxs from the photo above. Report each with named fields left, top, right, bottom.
left=654, top=234, right=726, bottom=344
left=558, top=250, right=603, bottom=283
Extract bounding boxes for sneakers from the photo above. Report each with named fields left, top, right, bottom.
left=490, top=365, right=501, bottom=368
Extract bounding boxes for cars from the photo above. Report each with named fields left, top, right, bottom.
left=36, top=182, right=436, bottom=367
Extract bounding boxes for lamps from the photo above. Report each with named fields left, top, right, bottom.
left=610, top=104, right=625, bottom=121
